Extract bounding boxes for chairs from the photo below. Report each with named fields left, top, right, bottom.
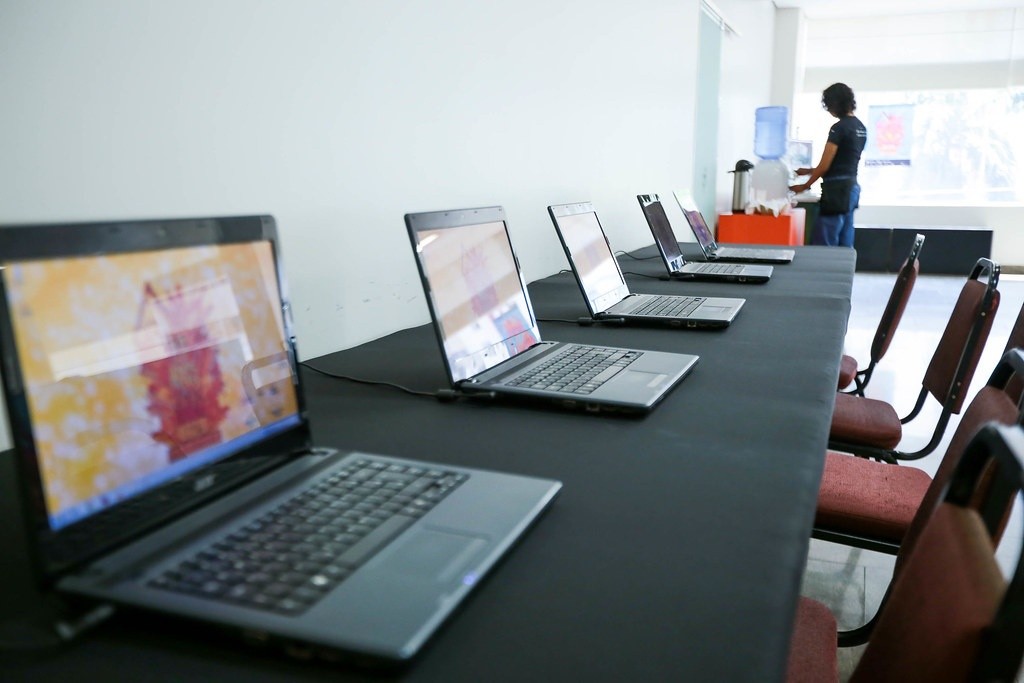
left=809, top=302, right=1024, bottom=558
left=783, top=349, right=1024, bottom=682
left=836, top=233, right=925, bottom=399
left=829, top=259, right=1001, bottom=465
left=842, top=422, right=1024, bottom=683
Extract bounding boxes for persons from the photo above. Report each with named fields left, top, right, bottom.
left=788, top=83, right=867, bottom=247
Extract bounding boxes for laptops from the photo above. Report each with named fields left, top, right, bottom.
left=547, top=202, right=747, bottom=328
left=403, top=206, right=699, bottom=410
left=636, top=194, right=774, bottom=281
left=673, top=191, right=796, bottom=261
left=0, top=215, right=562, bottom=659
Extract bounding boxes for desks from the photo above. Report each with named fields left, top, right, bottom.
left=0, top=242, right=857, bottom=683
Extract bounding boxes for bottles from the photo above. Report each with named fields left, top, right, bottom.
left=755, top=105, right=788, bottom=159
left=727, top=160, right=754, bottom=215
